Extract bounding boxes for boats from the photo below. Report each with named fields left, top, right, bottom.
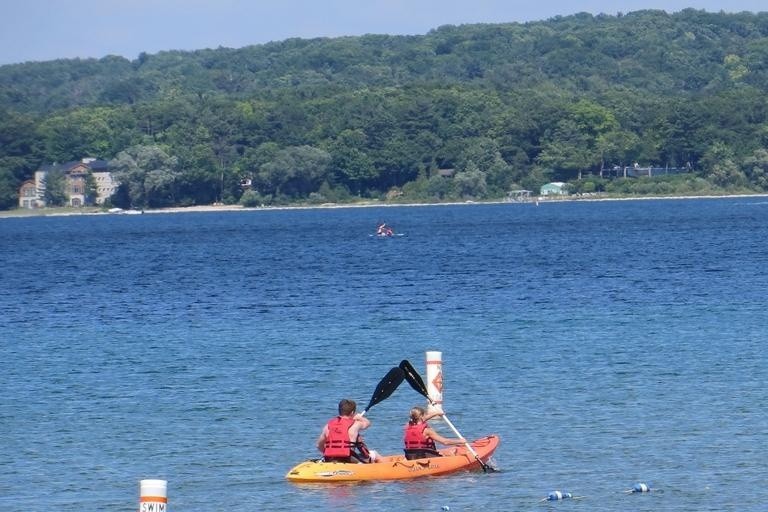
left=285, top=434, right=500, bottom=483
left=369, top=234, right=405, bottom=237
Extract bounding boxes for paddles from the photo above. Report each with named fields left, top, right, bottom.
left=360, top=368, right=404, bottom=417
left=400, top=359, right=495, bottom=474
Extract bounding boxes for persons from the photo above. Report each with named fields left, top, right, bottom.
left=316, top=399, right=384, bottom=464
left=401, top=407, right=466, bottom=462
left=376, top=223, right=386, bottom=236
